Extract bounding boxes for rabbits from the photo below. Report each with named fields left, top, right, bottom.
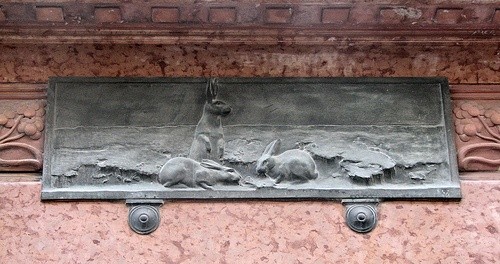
left=256, top=140, right=318, bottom=184
left=158, top=157, right=242, bottom=189
left=189, top=78, right=232, bottom=164
